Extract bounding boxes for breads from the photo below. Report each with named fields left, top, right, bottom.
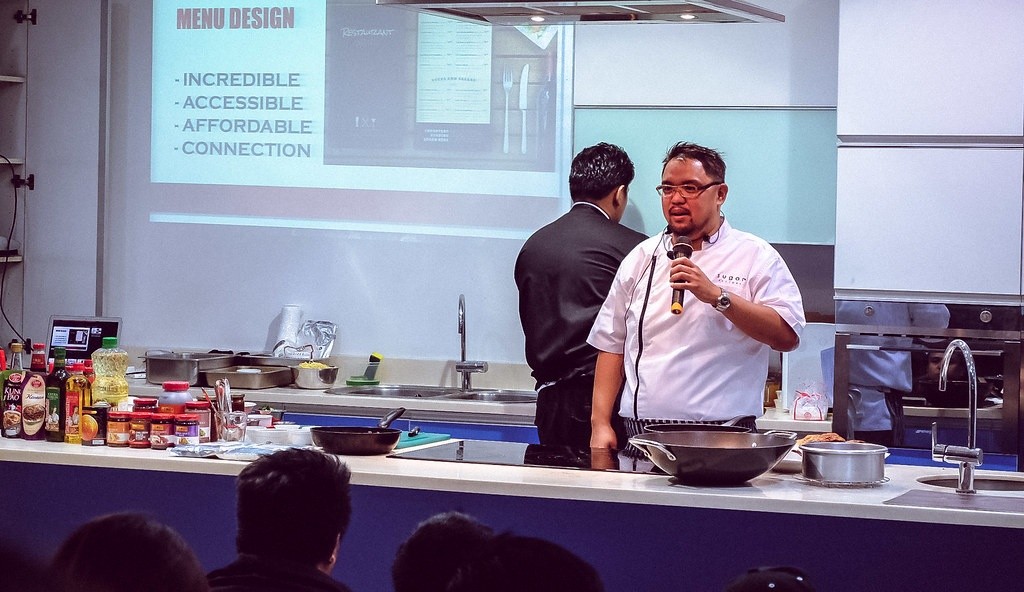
left=797, top=433, right=863, bottom=444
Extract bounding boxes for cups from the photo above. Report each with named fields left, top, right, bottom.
left=774, top=390, right=782, bottom=408
left=215, top=410, right=245, bottom=444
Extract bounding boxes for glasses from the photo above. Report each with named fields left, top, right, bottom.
left=656, top=180, right=724, bottom=198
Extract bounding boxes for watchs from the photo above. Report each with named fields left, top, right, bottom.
left=713, top=287, right=731, bottom=313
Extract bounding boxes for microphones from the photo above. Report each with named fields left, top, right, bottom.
left=670, top=236, right=694, bottom=314
left=703, top=228, right=720, bottom=245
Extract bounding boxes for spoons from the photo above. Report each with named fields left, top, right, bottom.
left=201, top=378, right=243, bottom=441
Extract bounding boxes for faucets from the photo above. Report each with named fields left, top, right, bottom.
left=455, top=293, right=499, bottom=393
left=929, top=337, right=984, bottom=495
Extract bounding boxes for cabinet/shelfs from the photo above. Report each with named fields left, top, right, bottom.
left=0, top=0, right=107, bottom=366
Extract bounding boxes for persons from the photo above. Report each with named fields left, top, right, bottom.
left=590, top=140, right=805, bottom=449
left=720, top=566, right=818, bottom=592
left=207, top=448, right=355, bottom=592
left=835, top=301, right=950, bottom=448
left=48, top=509, right=211, bottom=592
left=0, top=539, right=63, bottom=592
left=916, top=352, right=974, bottom=406
left=514, top=143, right=649, bottom=428
left=390, top=510, right=606, bottom=591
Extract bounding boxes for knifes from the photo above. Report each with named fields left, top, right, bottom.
left=518, top=63, right=529, bottom=154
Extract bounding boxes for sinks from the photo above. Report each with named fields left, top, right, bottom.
left=916, top=474, right=1024, bottom=492
left=323, top=383, right=454, bottom=397
left=449, top=390, right=538, bottom=404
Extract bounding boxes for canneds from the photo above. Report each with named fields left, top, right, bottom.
left=763, top=379, right=779, bottom=408
left=81, top=394, right=246, bottom=450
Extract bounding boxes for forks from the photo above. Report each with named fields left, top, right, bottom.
left=503, top=64, right=513, bottom=154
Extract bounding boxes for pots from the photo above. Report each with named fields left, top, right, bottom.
left=310, top=407, right=407, bottom=456
left=977, top=377, right=1003, bottom=399
left=627, top=429, right=798, bottom=486
left=917, top=378, right=994, bottom=408
left=643, top=415, right=756, bottom=433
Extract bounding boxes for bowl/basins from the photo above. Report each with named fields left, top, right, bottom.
left=287, top=365, right=340, bottom=390
left=790, top=442, right=888, bottom=482
left=244, top=401, right=321, bottom=445
left=343, top=360, right=384, bottom=385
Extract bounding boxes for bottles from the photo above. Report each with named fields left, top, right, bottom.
left=0, top=336, right=129, bottom=445
left=157, top=380, right=194, bottom=414
